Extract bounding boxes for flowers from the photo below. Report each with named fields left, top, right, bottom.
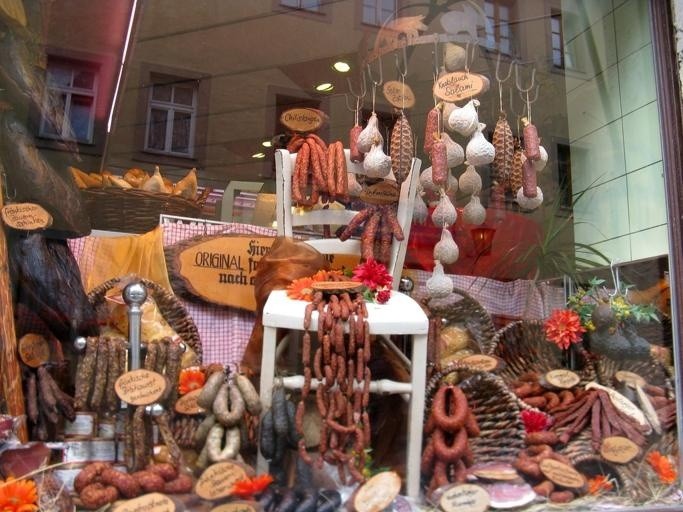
left=231, top=473, right=273, bottom=497
left=541, top=277, right=631, bottom=351
left=0, top=475, right=41, bottom=512
left=624, top=302, right=664, bottom=325
left=588, top=474, right=614, bottom=497
left=647, top=450, right=678, bottom=501
left=179, top=369, right=206, bottom=395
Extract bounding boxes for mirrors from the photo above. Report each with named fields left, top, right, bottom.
left=566, top=254, right=676, bottom=395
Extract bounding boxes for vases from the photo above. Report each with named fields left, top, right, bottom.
left=590, top=327, right=632, bottom=358
left=623, top=325, right=652, bottom=361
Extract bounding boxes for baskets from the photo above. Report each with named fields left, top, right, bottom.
left=77, top=186, right=212, bottom=234
left=421, top=283, right=672, bottom=482
left=86, top=276, right=201, bottom=366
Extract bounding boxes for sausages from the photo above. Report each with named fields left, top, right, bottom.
left=24, top=104, right=679, bottom=507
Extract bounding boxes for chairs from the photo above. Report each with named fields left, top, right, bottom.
left=255, top=147, right=429, bottom=498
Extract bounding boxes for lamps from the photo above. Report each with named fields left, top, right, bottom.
left=471, top=222, right=496, bottom=257
